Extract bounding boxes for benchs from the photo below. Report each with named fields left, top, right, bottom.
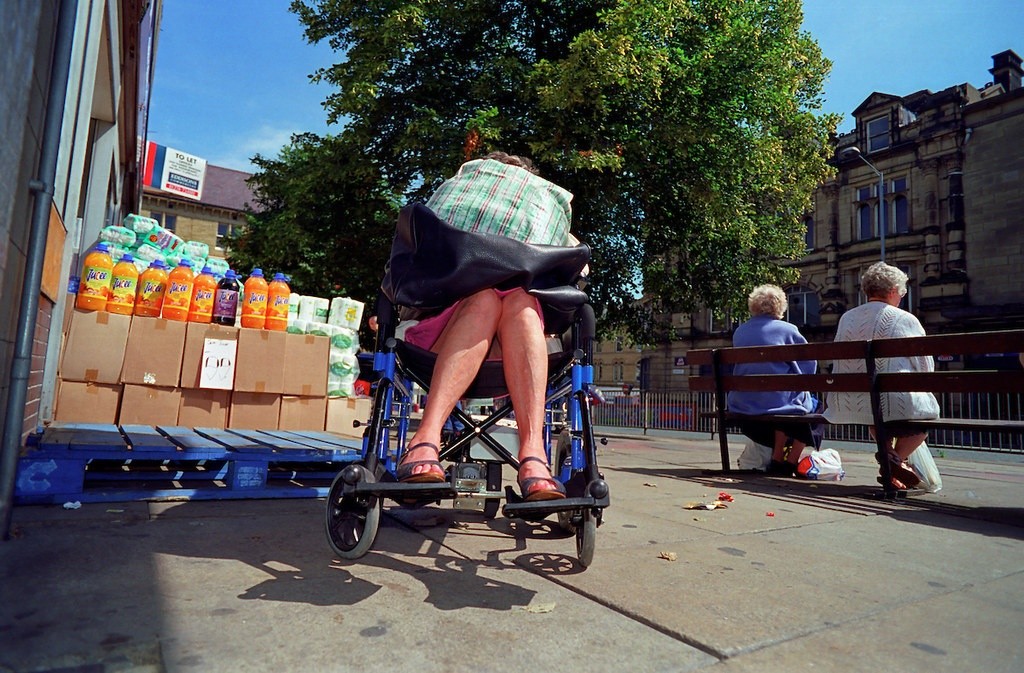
left=687, top=331, right=1024, bottom=501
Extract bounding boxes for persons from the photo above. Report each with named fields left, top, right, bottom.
left=823, top=262, right=940, bottom=491
left=725, top=283, right=818, bottom=475
left=366, top=150, right=592, bottom=501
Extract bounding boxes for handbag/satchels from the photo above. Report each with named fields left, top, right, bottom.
left=380, top=201, right=593, bottom=334
left=738, top=442, right=773, bottom=472
left=797, top=448, right=845, bottom=480
left=908, top=441, right=942, bottom=494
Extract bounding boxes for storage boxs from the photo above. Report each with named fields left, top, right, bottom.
left=283, top=333, right=332, bottom=397
left=117, top=383, right=183, bottom=430
left=60, top=292, right=77, bottom=333
left=324, top=395, right=373, bottom=439
left=181, top=321, right=240, bottom=392
left=177, top=388, right=232, bottom=431
left=60, top=307, right=132, bottom=387
left=227, top=391, right=282, bottom=431
left=278, top=395, right=327, bottom=431
left=54, top=381, right=123, bottom=424
left=232, top=327, right=287, bottom=394
left=120, top=314, right=188, bottom=388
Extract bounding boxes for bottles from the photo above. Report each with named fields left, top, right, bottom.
left=188, top=266, right=217, bottom=324
left=211, top=269, right=240, bottom=327
left=162, top=259, right=194, bottom=321
left=105, top=253, right=139, bottom=315
left=134, top=259, right=167, bottom=317
left=264, top=272, right=290, bottom=331
left=240, top=268, right=269, bottom=329
left=76, top=243, right=113, bottom=310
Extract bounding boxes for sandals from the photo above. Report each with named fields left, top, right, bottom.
left=516, top=456, right=568, bottom=501
left=395, top=442, right=447, bottom=482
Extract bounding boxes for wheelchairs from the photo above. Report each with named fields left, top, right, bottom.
left=324, top=278, right=610, bottom=568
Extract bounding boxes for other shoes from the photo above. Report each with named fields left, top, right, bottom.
left=874, top=450, right=919, bottom=488
left=777, top=461, right=800, bottom=478
left=766, top=460, right=780, bottom=476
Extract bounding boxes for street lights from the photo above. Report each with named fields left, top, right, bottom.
left=840, top=145, right=885, bottom=264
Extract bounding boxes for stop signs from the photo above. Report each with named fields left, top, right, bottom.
left=622, top=384, right=630, bottom=395
